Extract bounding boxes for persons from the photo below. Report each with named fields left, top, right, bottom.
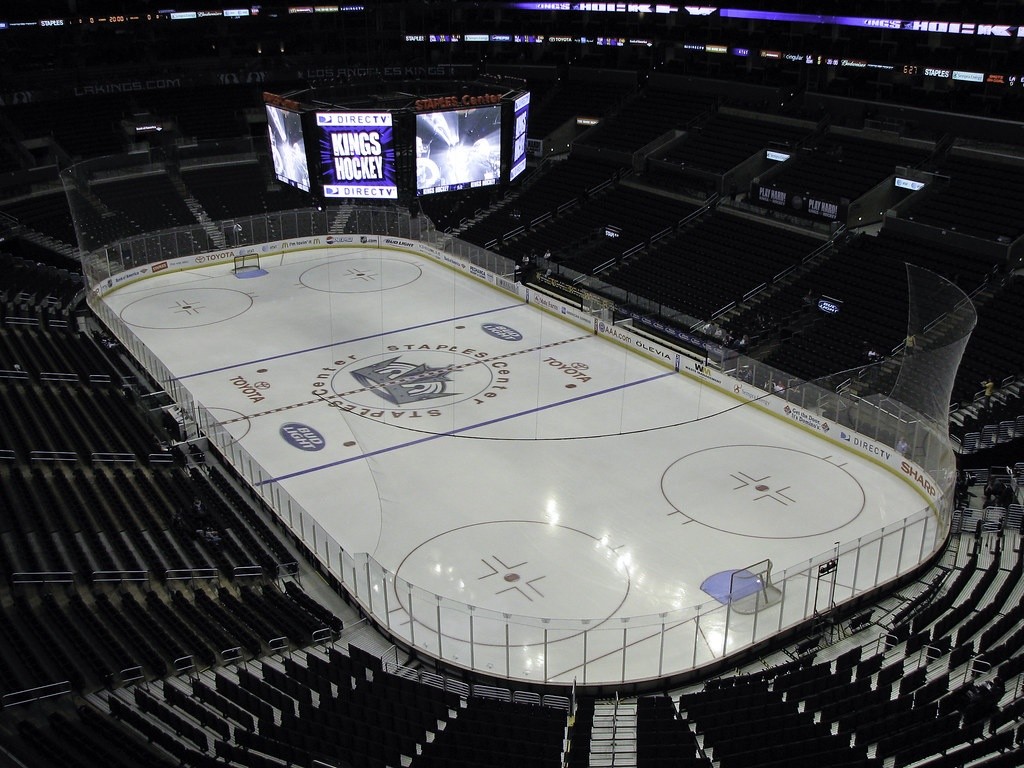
left=903, top=331, right=916, bottom=356
left=173, top=494, right=223, bottom=548
left=514, top=247, right=554, bottom=283
left=896, top=437, right=908, bottom=455
left=981, top=377, right=994, bottom=409
left=699, top=319, right=751, bottom=355
left=950, top=467, right=1013, bottom=510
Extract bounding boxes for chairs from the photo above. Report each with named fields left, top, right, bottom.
left=0, top=50, right=1024, bottom=768
